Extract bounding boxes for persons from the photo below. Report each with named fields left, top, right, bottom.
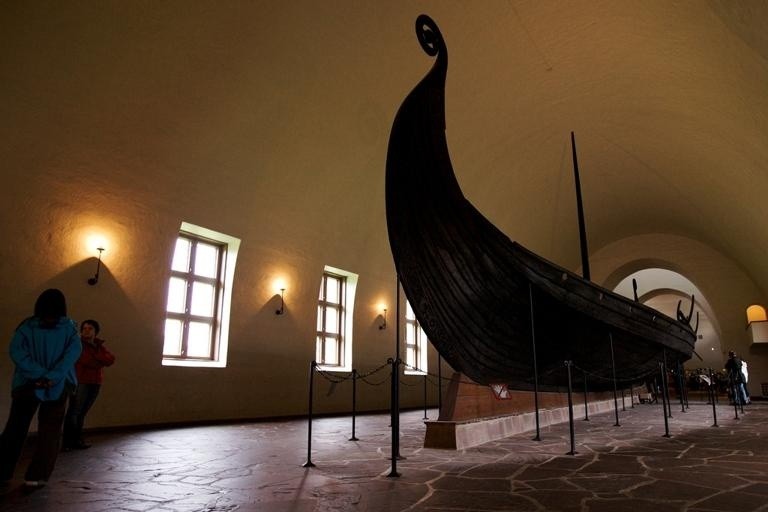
left=66, top=319, right=115, bottom=451
left=724, top=350, right=750, bottom=405
left=0, top=288, right=83, bottom=492
left=734, top=358, right=749, bottom=404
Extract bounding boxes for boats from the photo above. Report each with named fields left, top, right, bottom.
left=384, top=13, right=701, bottom=394
